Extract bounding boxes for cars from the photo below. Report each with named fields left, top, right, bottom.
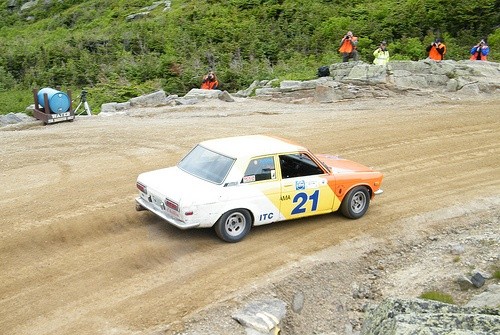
left=134, top=133, right=384, bottom=243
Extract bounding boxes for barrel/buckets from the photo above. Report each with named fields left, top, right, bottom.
left=37, top=87, right=70, bottom=113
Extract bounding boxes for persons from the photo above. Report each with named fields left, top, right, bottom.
left=201, top=72, right=218, bottom=90
left=426, top=37, right=446, bottom=60
left=373, top=42, right=389, bottom=65
left=338, top=31, right=358, bottom=62
left=469, top=38, right=489, bottom=61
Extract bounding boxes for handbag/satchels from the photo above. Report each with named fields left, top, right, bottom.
left=318, top=67, right=329, bottom=77
